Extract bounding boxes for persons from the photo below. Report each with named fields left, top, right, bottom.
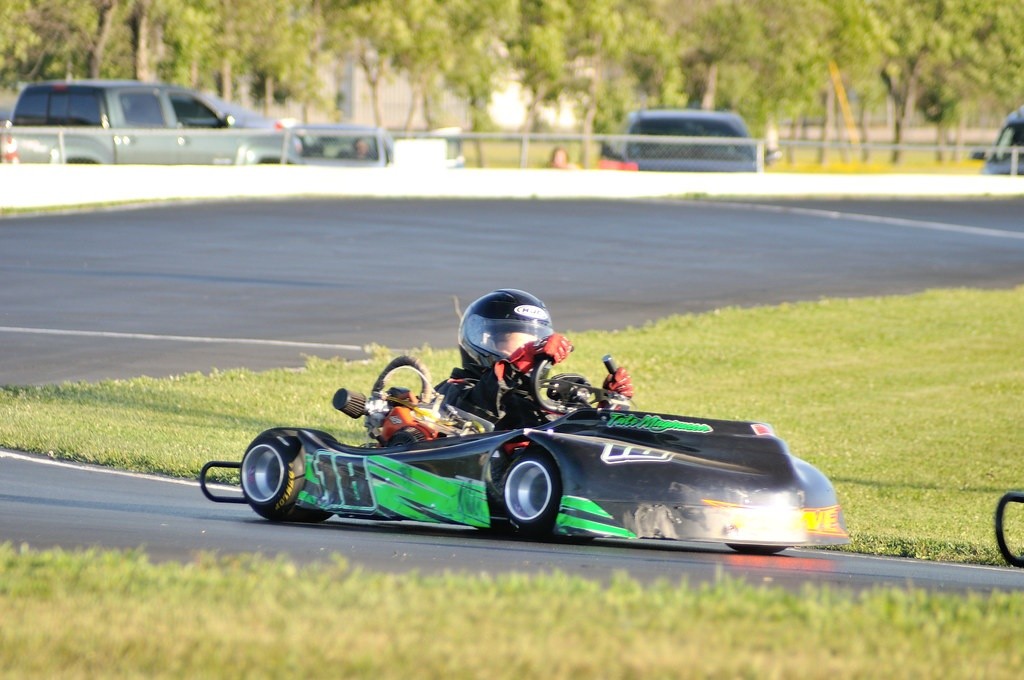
left=353, top=139, right=373, bottom=161
left=431, top=289, right=633, bottom=432
left=545, top=148, right=570, bottom=168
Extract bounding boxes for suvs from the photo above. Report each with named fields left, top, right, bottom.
left=1, top=81, right=394, bottom=167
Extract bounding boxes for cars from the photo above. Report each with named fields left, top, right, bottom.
left=971, top=103, right=1024, bottom=176
left=599, top=107, right=778, bottom=170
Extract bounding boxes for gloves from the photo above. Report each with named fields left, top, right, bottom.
left=525, top=332, right=574, bottom=365
left=597, top=368, right=633, bottom=410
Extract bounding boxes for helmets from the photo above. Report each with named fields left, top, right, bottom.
left=458, top=288, right=555, bottom=388
